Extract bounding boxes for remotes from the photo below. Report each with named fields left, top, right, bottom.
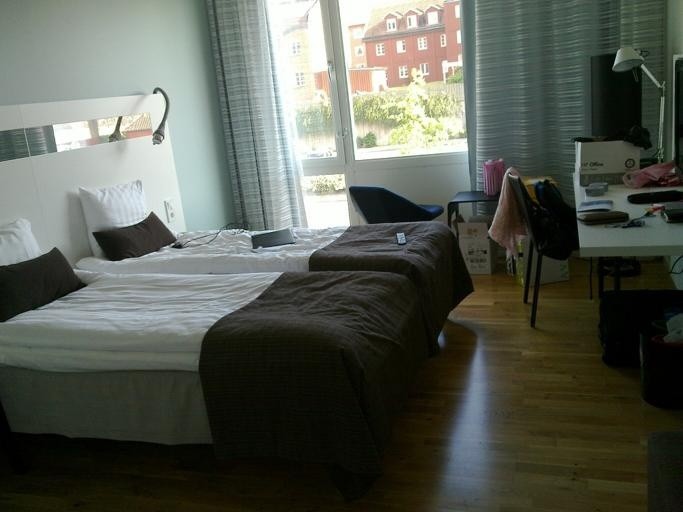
left=396, top=232, right=406, bottom=245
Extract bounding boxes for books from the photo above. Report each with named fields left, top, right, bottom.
left=661, top=208, right=683, bottom=223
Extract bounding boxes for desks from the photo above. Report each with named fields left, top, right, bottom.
left=449, top=191, right=498, bottom=228
left=573, top=172, right=683, bottom=258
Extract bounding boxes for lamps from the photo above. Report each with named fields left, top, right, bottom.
left=153, top=87, right=170, bottom=145
left=613, top=47, right=666, bottom=163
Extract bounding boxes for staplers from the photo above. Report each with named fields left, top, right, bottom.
left=640, top=157, right=664, bottom=169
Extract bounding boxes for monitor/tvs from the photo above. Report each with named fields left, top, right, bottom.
left=673, top=57, right=683, bottom=182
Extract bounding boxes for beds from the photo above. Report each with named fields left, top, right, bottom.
left=0, top=269, right=440, bottom=501
left=74, top=221, right=475, bottom=333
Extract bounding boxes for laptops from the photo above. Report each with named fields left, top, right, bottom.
left=252, top=228, right=296, bottom=249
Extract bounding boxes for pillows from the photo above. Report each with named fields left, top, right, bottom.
left=0, top=218, right=41, bottom=265
left=0, top=247, right=87, bottom=322
left=79, top=180, right=146, bottom=258
left=93, top=213, right=177, bottom=261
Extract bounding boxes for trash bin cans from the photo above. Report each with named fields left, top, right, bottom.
left=639, top=325, right=683, bottom=410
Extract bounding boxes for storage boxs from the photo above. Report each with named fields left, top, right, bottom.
left=575, top=141, right=641, bottom=186
left=453, top=215, right=497, bottom=275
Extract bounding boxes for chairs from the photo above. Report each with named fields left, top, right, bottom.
left=508, top=173, right=620, bottom=328
left=350, top=187, right=444, bottom=224
